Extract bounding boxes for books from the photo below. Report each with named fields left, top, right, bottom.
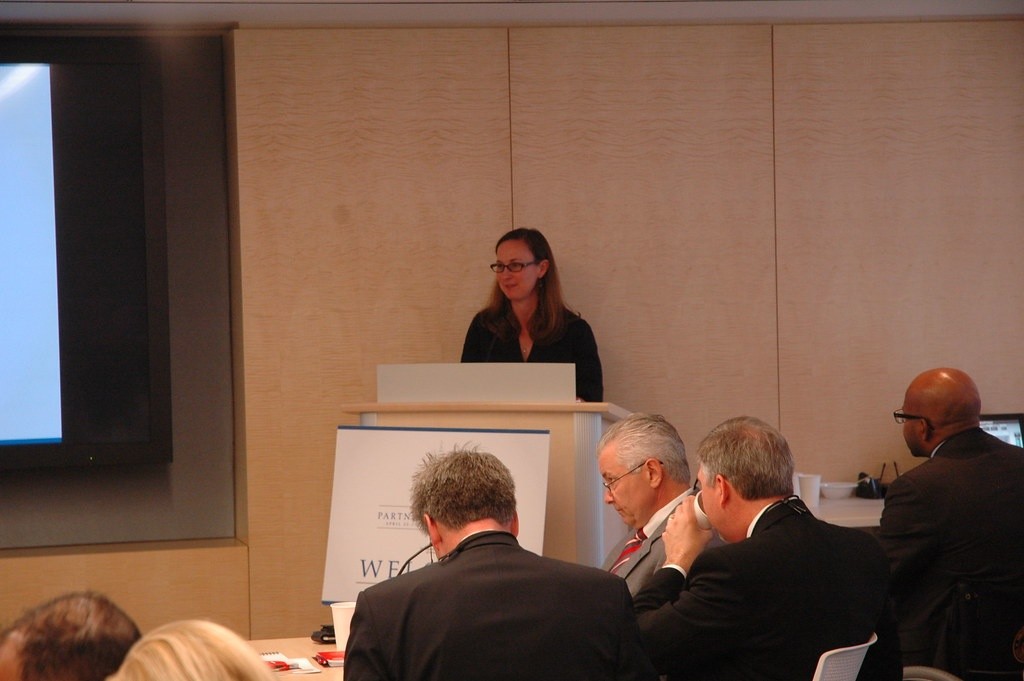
left=316, top=651, right=345, bottom=667
left=260, top=652, right=301, bottom=672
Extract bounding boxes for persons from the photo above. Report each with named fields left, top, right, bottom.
left=876, top=366, right=1024, bottom=681
left=0, top=591, right=279, bottom=681
left=342, top=450, right=655, bottom=681
left=632, top=417, right=885, bottom=681
left=461, top=227, right=603, bottom=403
left=594, top=414, right=721, bottom=598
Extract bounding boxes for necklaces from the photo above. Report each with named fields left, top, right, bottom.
left=519, top=340, right=532, bottom=353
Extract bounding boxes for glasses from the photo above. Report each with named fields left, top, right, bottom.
left=602, top=456, right=664, bottom=494
left=489, top=259, right=537, bottom=273
left=893, top=409, right=931, bottom=428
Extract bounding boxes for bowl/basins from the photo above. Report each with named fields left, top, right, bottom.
left=820, top=482, right=859, bottom=500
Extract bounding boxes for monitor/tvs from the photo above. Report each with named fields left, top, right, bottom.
left=979, top=413, right=1024, bottom=449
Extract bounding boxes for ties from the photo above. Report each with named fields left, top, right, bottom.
left=607, top=525, right=647, bottom=575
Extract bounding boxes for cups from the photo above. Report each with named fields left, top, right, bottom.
left=693, top=491, right=713, bottom=530
left=331, top=602, right=356, bottom=651
left=799, top=474, right=821, bottom=507
